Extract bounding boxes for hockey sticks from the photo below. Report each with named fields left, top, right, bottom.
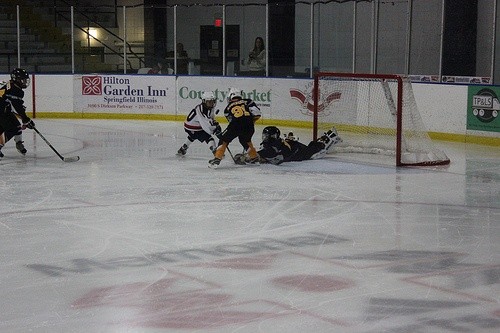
left=227, top=145, right=247, bottom=165
left=32, top=126, right=80, bottom=163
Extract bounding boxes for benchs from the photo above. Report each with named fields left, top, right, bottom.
left=0, top=1, right=119, bottom=75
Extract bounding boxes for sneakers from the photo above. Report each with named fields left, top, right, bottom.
left=0, top=148, right=5, bottom=162
left=176, top=146, right=187, bottom=159
left=16, top=141, right=27, bottom=156
left=212, top=150, right=226, bottom=161
left=244, top=156, right=261, bottom=166
left=207, top=157, right=221, bottom=169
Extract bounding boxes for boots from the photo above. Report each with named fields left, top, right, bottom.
left=287, top=132, right=299, bottom=142
left=316, top=126, right=343, bottom=155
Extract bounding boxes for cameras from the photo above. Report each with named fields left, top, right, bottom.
left=249, top=54, right=253, bottom=58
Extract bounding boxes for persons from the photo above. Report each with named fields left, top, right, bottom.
left=0, top=67, right=35, bottom=157
left=208, top=87, right=262, bottom=169
left=167, top=42, right=188, bottom=57
left=175, top=91, right=225, bottom=159
left=247, top=36, right=266, bottom=76
left=149, top=39, right=166, bottom=69
left=234, top=126, right=343, bottom=166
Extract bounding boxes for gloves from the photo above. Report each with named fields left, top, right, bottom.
left=233, top=153, right=245, bottom=164
left=213, top=120, right=219, bottom=126
left=22, top=115, right=35, bottom=130
left=216, top=125, right=221, bottom=134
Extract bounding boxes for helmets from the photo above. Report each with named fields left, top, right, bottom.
left=261, top=126, right=280, bottom=143
left=10, top=68, right=30, bottom=89
left=201, top=90, right=217, bottom=110
left=226, top=89, right=243, bottom=101
